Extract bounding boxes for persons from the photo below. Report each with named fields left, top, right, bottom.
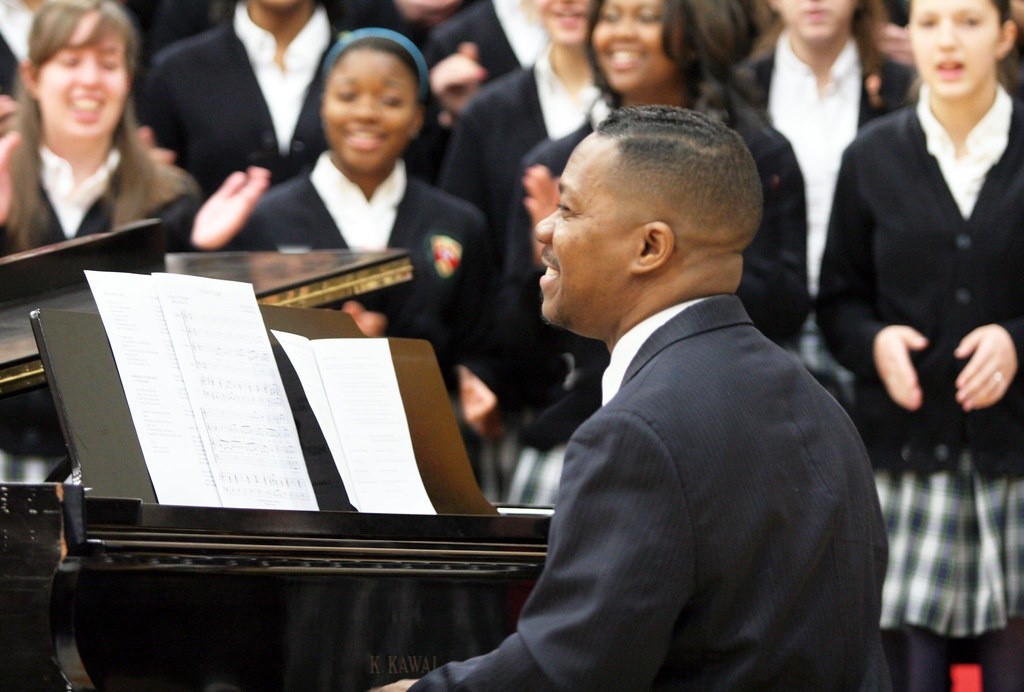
left=0, top=2, right=1024, bottom=514
left=357, top=100, right=901, bottom=692
left=813, top=0, right=1024, bottom=692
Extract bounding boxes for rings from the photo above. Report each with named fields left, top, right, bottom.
left=996, top=371, right=1005, bottom=388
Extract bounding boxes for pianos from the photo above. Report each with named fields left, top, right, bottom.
left=0, top=216, right=555, bottom=692
